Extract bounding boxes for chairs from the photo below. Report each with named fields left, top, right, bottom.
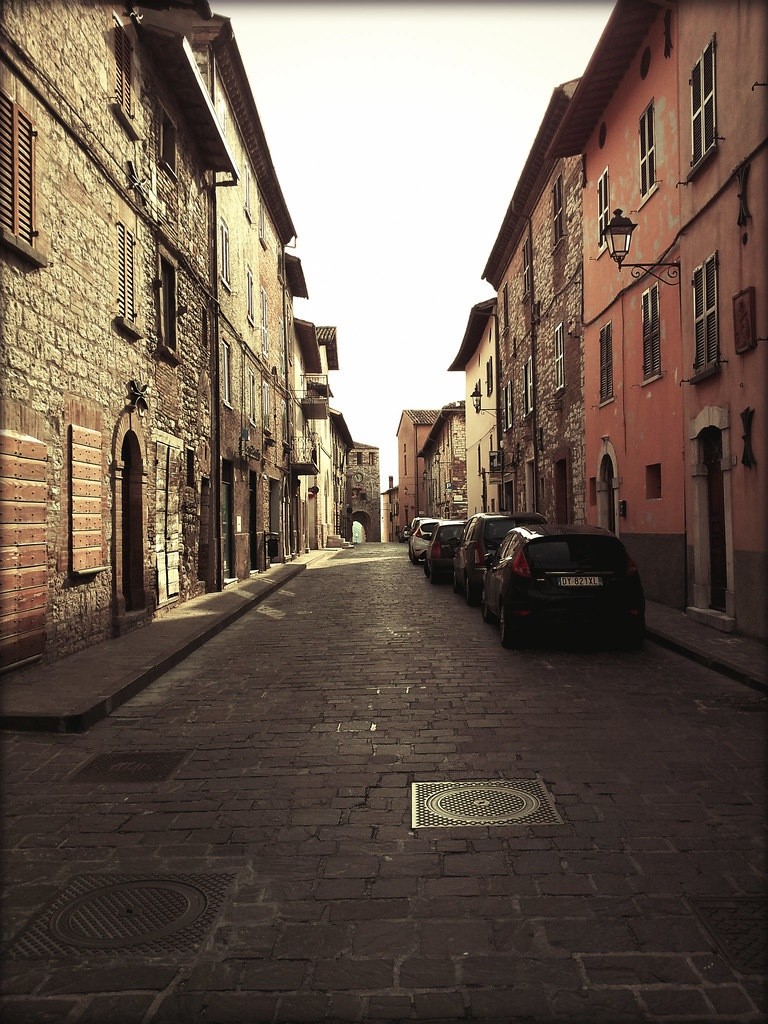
left=444, top=529, right=456, bottom=539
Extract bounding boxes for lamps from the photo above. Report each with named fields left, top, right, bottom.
left=403, top=487, right=414, bottom=495
left=469, top=388, right=504, bottom=421
left=422, top=469, right=437, bottom=481
left=600, top=209, right=681, bottom=286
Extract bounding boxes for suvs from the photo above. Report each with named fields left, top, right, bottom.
left=407, top=517, right=450, bottom=566
left=423, top=521, right=467, bottom=584
left=401, top=526, right=411, bottom=542
left=448, top=512, right=548, bottom=607
left=482, top=524, right=646, bottom=647
